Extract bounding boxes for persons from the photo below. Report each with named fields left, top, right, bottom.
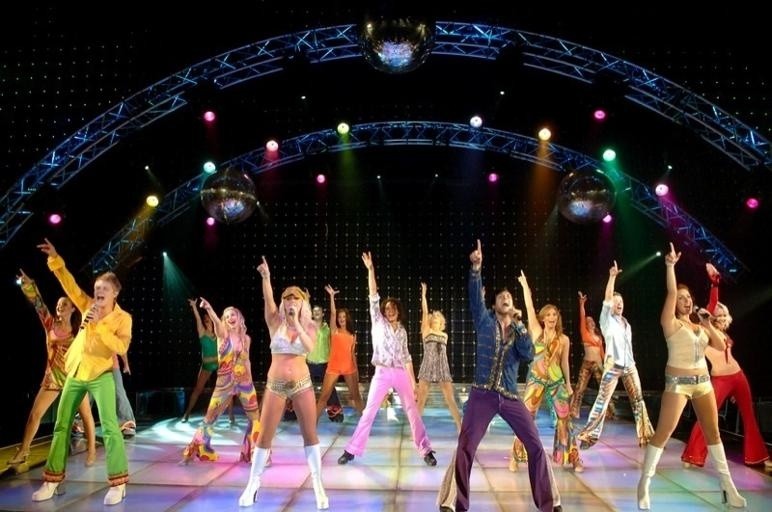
left=510, top=242, right=772, bottom=510
left=177, top=251, right=462, bottom=509
left=437, top=240, right=563, bottom=512
left=3, top=237, right=136, bottom=505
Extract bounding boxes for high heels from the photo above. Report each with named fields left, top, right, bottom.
left=8, top=451, right=30, bottom=464
left=85, top=453, right=96, bottom=466
left=104, top=483, right=126, bottom=505
left=32, top=481, right=65, bottom=501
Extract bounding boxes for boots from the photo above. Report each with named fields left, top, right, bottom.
left=638, top=443, right=664, bottom=509
left=304, top=443, right=330, bottom=509
left=707, top=441, right=746, bottom=507
left=238, top=447, right=270, bottom=506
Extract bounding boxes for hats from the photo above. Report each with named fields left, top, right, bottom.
left=283, top=287, right=306, bottom=299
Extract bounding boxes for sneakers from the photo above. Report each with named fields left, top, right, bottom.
left=424, top=453, right=437, bottom=466
left=338, top=451, right=354, bottom=464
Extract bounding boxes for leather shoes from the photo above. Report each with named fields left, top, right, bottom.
left=573, top=463, right=583, bottom=473
left=764, top=461, right=772, bottom=468
left=509, top=461, right=519, bottom=472
left=683, top=462, right=691, bottom=469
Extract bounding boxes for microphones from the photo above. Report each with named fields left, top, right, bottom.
left=80, top=304, right=97, bottom=329
left=509, top=307, right=522, bottom=320
left=693, top=306, right=709, bottom=319
left=289, top=308, right=294, bottom=315
